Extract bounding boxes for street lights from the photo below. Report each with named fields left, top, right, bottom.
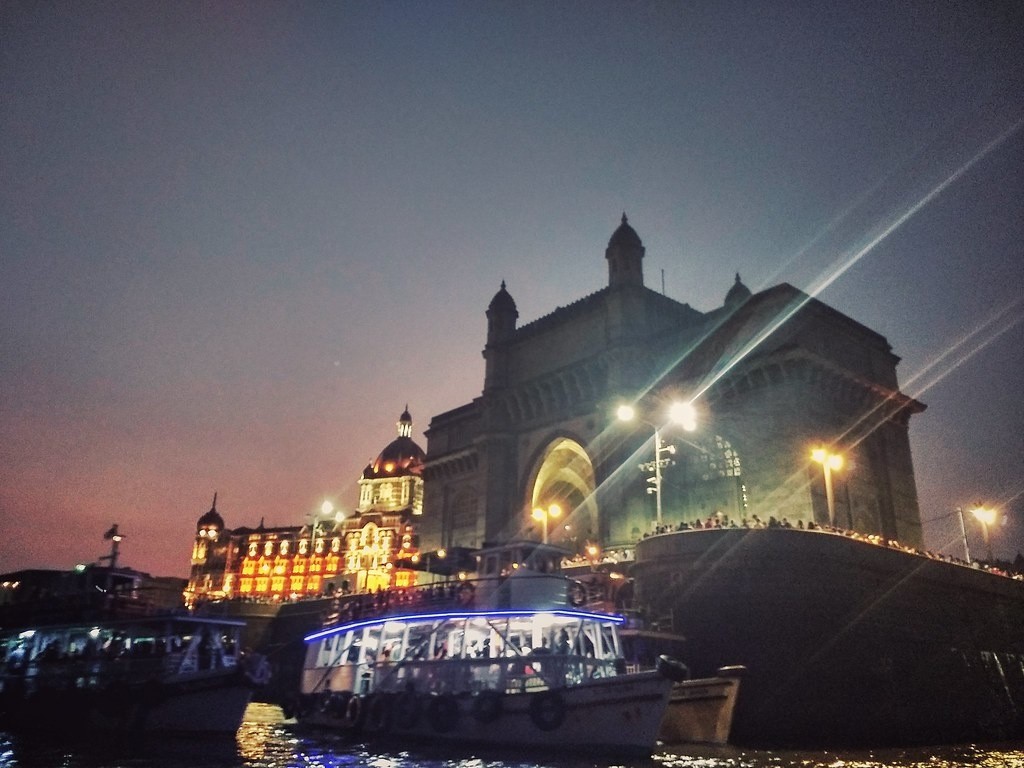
left=971, top=504, right=1003, bottom=567
left=605, top=391, right=699, bottom=529
left=806, top=444, right=847, bottom=530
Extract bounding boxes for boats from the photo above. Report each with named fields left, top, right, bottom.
left=288, top=539, right=686, bottom=758
left=3, top=603, right=268, bottom=737
left=660, top=661, right=746, bottom=748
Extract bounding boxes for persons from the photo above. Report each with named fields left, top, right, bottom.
left=4, top=626, right=224, bottom=671
left=560, top=548, right=637, bottom=568
left=643, top=511, right=1024, bottom=581
left=360, top=629, right=596, bottom=691
left=223, top=583, right=433, bottom=607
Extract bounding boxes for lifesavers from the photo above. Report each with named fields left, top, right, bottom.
left=566, top=580, right=589, bottom=608
left=456, top=582, right=475, bottom=607
